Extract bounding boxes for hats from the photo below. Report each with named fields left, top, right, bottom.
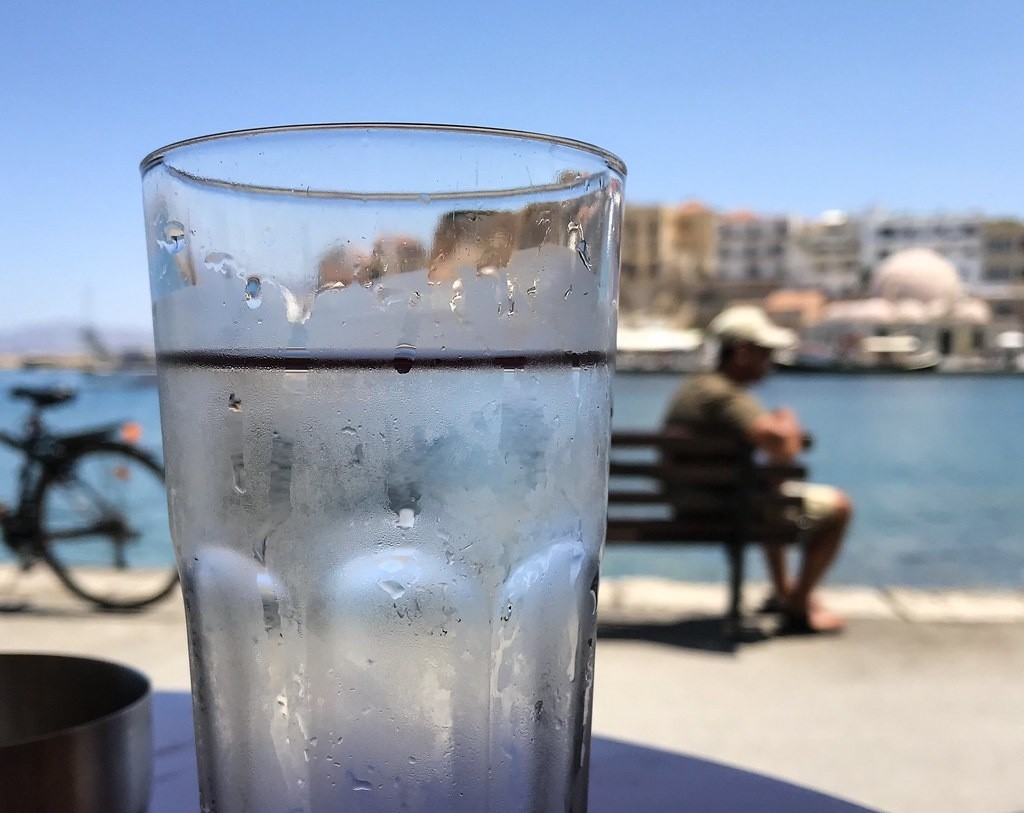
left=712, top=305, right=796, bottom=349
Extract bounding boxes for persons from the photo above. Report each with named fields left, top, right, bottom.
left=655, top=301, right=850, bottom=637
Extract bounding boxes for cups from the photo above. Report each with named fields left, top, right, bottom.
left=141, top=120, right=625, bottom=813
left=0, top=652, right=152, bottom=813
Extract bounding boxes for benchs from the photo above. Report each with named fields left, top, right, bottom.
left=605, top=430, right=816, bottom=649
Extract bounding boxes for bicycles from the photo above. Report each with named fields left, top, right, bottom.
left=0, top=385, right=181, bottom=611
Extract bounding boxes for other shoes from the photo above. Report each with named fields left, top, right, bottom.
left=765, top=595, right=821, bottom=611
left=782, top=611, right=845, bottom=633
